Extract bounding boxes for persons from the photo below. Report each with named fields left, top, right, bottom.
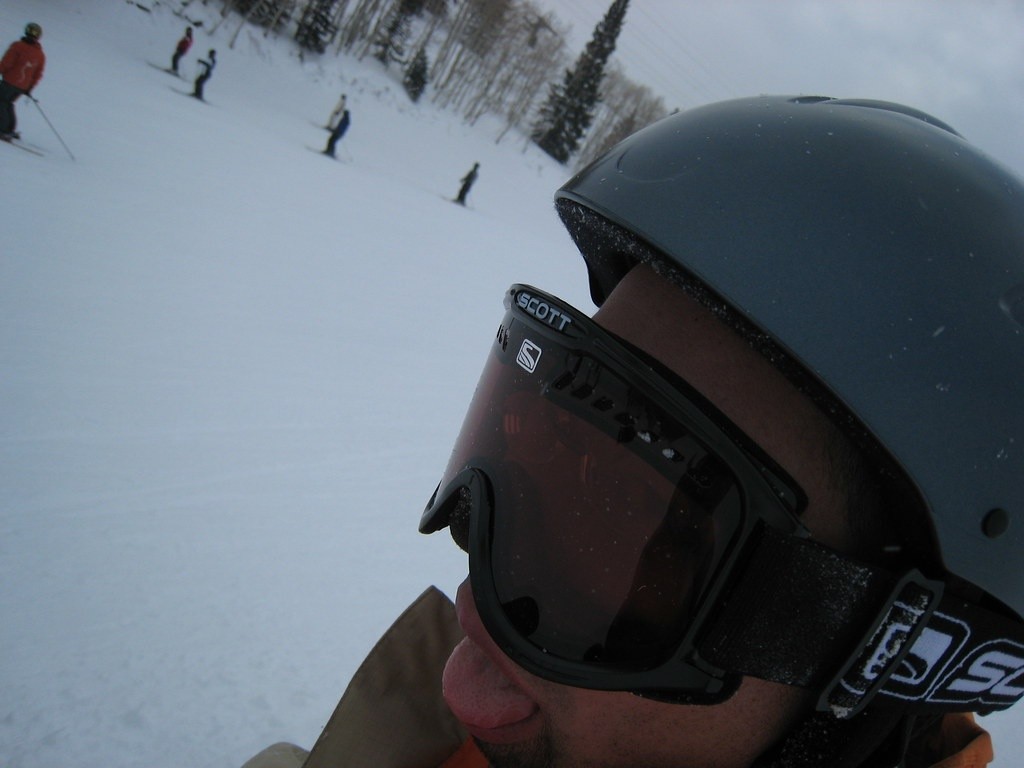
left=323, top=109, right=350, bottom=158
left=453, top=162, right=479, bottom=205
left=0, top=22, right=45, bottom=143
left=165, top=26, right=194, bottom=76
left=323, top=94, right=346, bottom=131
left=192, top=50, right=217, bottom=98
left=236, top=94, right=1024, bottom=768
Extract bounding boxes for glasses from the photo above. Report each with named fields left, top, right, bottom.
left=421, top=284, right=1024, bottom=718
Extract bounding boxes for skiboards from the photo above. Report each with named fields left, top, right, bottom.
left=5, top=137, right=49, bottom=158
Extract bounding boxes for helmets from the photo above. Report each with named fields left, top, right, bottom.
left=26, top=23, right=42, bottom=39
left=553, top=94, right=1024, bottom=620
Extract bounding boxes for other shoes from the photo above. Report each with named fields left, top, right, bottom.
left=7, top=128, right=15, bottom=135
left=0, top=130, right=9, bottom=138
